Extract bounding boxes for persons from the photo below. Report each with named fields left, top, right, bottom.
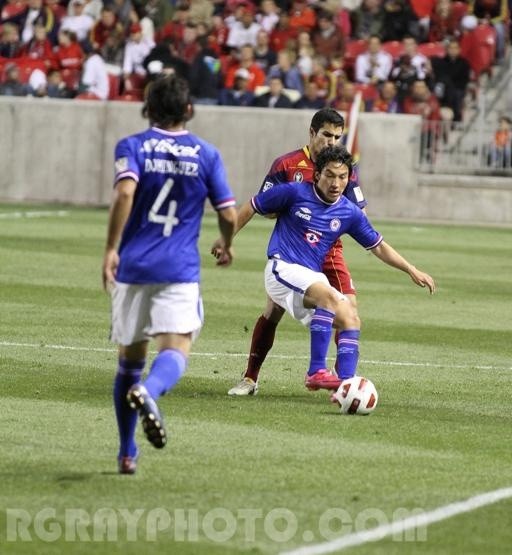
left=98, top=72, right=238, bottom=476
left=210, top=144, right=437, bottom=405
left=0, top=0, right=512, bottom=179
left=225, top=109, right=372, bottom=397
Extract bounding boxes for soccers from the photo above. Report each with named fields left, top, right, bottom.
left=336, top=376, right=378, bottom=415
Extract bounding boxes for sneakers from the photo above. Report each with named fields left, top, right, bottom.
left=128, top=383, right=168, bottom=449
left=119, top=442, right=140, bottom=473
left=304, top=368, right=342, bottom=391
left=227, top=377, right=258, bottom=396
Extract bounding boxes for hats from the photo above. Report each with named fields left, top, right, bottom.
left=129, top=24, right=141, bottom=33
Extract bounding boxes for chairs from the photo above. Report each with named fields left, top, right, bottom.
left=76, top=8, right=497, bottom=118
